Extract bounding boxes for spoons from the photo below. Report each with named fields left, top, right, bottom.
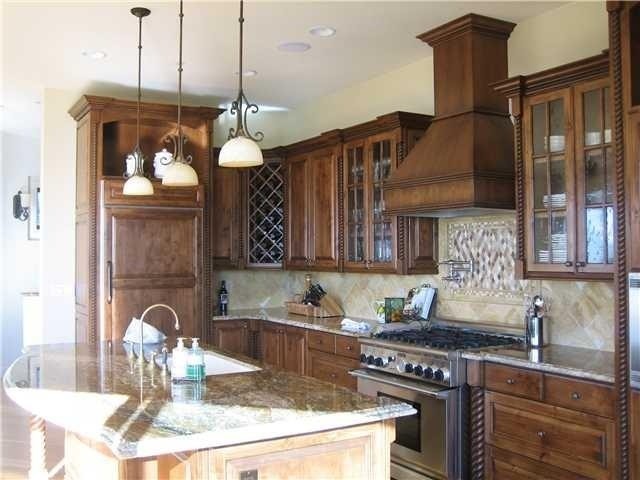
left=532, top=295, right=544, bottom=315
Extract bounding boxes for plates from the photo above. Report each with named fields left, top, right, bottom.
left=123, top=336, right=167, bottom=344
left=541, top=192, right=567, bottom=210
left=538, top=232, right=568, bottom=264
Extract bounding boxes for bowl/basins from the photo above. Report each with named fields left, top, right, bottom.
left=544, top=129, right=613, bottom=152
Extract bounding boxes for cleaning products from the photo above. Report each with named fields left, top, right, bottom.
left=187, top=337, right=205, bottom=380
left=187, top=380, right=206, bottom=406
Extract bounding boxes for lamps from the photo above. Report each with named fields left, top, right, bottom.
left=162, top=0, right=198, bottom=188
left=121, top=7, right=155, bottom=196
left=220, top=0, right=265, bottom=168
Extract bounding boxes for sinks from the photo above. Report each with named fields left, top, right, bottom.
left=154, top=349, right=264, bottom=378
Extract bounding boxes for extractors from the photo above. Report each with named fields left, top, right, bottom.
left=377, top=167, right=517, bottom=220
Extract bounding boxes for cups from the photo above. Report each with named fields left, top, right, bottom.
left=525, top=315, right=549, bottom=347
left=384, top=297, right=406, bottom=322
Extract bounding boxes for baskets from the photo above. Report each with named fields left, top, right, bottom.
left=287, top=300, right=316, bottom=316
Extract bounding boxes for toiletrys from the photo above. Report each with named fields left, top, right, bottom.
left=170, top=336, right=186, bottom=379
left=170, top=380, right=187, bottom=406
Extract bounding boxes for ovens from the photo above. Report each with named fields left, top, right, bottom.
left=347, top=369, right=470, bottom=480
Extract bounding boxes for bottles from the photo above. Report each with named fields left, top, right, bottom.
left=301, top=274, right=312, bottom=305
left=218, top=279, right=228, bottom=314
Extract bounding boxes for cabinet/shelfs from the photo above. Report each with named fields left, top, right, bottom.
left=253, top=318, right=307, bottom=381
left=212, top=146, right=286, bottom=269
left=68, top=94, right=228, bottom=345
left=286, top=130, right=343, bottom=271
left=189, top=418, right=396, bottom=480
left=343, top=112, right=437, bottom=274
left=487, top=49, right=614, bottom=280
left=308, top=327, right=361, bottom=394
left=463, top=353, right=640, bottom=480
left=214, top=319, right=253, bottom=358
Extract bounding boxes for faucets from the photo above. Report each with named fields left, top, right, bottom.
left=137, top=363, right=170, bottom=422
left=138, top=303, right=180, bottom=362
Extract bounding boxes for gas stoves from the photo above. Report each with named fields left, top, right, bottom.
left=356, top=327, right=524, bottom=387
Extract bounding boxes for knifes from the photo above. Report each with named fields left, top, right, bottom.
left=307, top=284, right=327, bottom=303
